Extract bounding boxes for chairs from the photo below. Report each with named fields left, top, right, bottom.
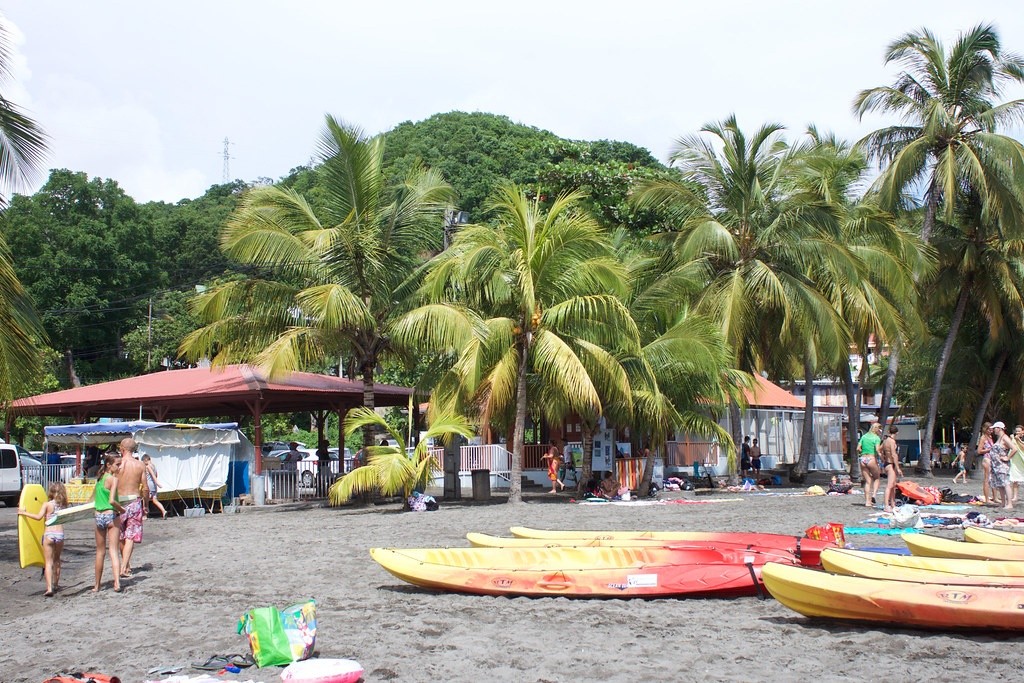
left=897, top=445, right=911, bottom=468
left=931, top=452, right=958, bottom=469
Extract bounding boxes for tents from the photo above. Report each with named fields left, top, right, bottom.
left=43, top=419, right=256, bottom=517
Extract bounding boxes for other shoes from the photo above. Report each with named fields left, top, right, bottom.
left=940, top=516, right=964, bottom=526
left=549, top=490, right=557, bottom=493
left=871, top=496, right=876, bottom=503
left=561, top=484, right=566, bottom=492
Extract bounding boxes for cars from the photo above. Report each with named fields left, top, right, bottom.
left=28, top=451, right=109, bottom=485
left=259, top=440, right=352, bottom=486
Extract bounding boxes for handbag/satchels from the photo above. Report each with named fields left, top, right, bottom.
left=237, top=599, right=318, bottom=668
left=893, top=504, right=925, bottom=530
left=938, top=487, right=952, bottom=503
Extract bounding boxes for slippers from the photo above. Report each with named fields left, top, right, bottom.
left=190, top=654, right=230, bottom=670
left=216, top=653, right=251, bottom=666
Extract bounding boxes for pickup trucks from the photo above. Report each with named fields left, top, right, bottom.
left=0, top=443, right=75, bottom=506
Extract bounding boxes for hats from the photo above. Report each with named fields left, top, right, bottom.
left=989, top=422, right=1006, bottom=430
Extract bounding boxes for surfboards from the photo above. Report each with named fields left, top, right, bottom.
left=17, top=483, right=49, bottom=569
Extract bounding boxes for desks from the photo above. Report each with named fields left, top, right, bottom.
left=61, top=484, right=227, bottom=516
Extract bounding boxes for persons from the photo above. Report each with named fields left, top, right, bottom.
left=540, top=438, right=649, bottom=502
left=741, top=436, right=751, bottom=477
left=115, top=439, right=150, bottom=578
left=18, top=482, right=69, bottom=596
left=91, top=453, right=126, bottom=592
left=41, top=446, right=167, bottom=521
left=283, top=440, right=388, bottom=497
left=749, top=439, right=761, bottom=482
left=877, top=421, right=1024, bottom=510
left=855, top=422, right=884, bottom=507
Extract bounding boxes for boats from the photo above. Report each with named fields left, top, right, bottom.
left=369, top=523, right=840, bottom=600
left=758, top=523, right=1023, bottom=634
